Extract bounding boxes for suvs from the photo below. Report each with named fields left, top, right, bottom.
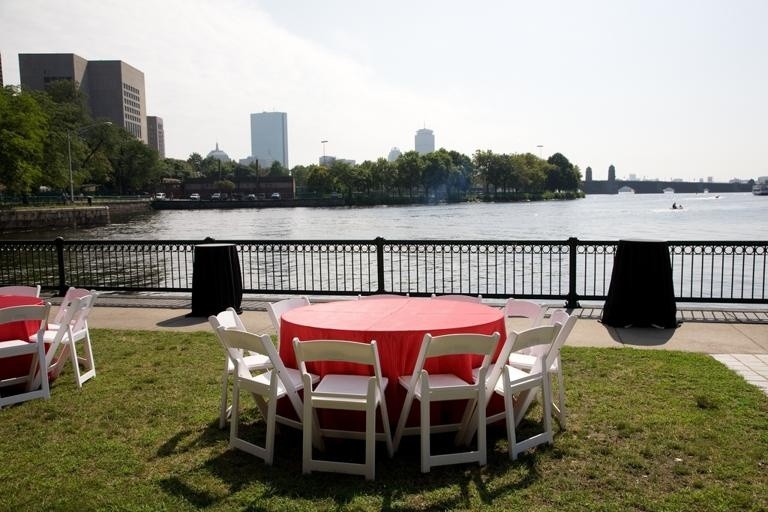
left=211, top=192, right=220, bottom=200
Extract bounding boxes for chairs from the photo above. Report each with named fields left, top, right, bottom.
left=264, top=296, right=310, bottom=331
left=358, top=293, right=409, bottom=300
left=0, top=285, right=41, bottom=297
left=0, top=301, right=51, bottom=407
left=393, top=331, right=501, bottom=472
left=217, top=325, right=320, bottom=467
left=208, top=307, right=284, bottom=437
left=32, top=292, right=99, bottom=390
left=502, top=297, right=548, bottom=332
left=292, top=337, right=393, bottom=481
left=432, top=293, right=482, bottom=305
left=509, top=310, right=578, bottom=428
left=462, top=321, right=562, bottom=462
left=47, top=287, right=99, bottom=370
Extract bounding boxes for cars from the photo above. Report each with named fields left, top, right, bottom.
left=271, top=193, right=280, bottom=199
left=247, top=194, right=255, bottom=200
left=189, top=193, right=200, bottom=199
left=154, top=192, right=165, bottom=198
left=259, top=193, right=265, bottom=198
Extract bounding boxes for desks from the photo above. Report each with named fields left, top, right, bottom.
left=0, top=295, right=58, bottom=382
left=280, top=298, right=506, bottom=444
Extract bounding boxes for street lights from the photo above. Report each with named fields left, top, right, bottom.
left=537, top=144, right=543, bottom=158
left=321, top=140, right=327, bottom=163
left=67, top=121, right=111, bottom=202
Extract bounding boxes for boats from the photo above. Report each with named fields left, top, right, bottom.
left=671, top=204, right=682, bottom=211
left=752, top=176, right=768, bottom=195
